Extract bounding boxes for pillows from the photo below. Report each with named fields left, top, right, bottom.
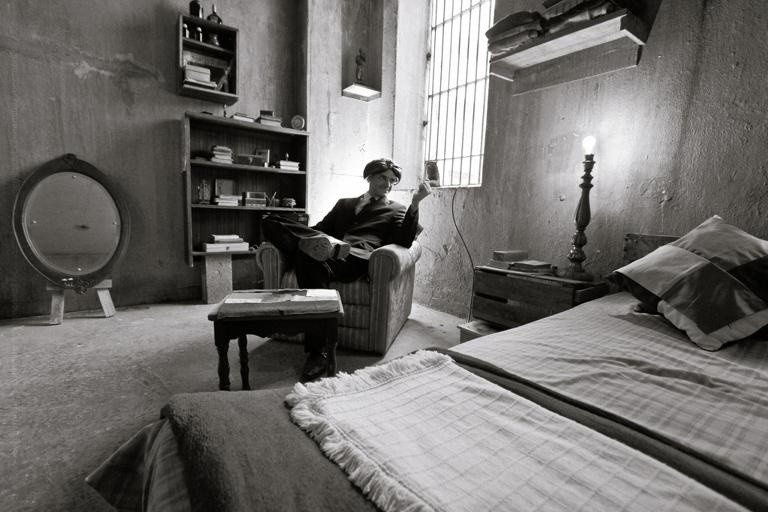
left=604, top=215, right=767, bottom=352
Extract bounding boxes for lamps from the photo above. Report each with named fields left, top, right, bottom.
left=556, top=135, right=596, bottom=282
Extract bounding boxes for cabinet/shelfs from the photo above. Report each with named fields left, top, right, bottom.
left=177, top=13, right=240, bottom=107
left=180, top=111, right=310, bottom=268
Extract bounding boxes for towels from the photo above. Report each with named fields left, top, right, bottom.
left=486, top=1, right=621, bottom=56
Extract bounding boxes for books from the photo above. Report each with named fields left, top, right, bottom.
left=486, top=249, right=551, bottom=274
left=183, top=64, right=301, bottom=253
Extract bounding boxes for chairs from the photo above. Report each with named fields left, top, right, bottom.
left=255, top=225, right=422, bottom=356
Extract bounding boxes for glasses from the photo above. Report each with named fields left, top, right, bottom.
left=377, top=174, right=399, bottom=185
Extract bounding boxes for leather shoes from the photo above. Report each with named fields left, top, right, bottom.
left=298, top=234, right=350, bottom=262
left=303, top=347, right=330, bottom=379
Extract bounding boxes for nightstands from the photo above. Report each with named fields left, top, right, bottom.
left=467, top=265, right=608, bottom=328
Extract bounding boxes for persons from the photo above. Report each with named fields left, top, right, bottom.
left=262, top=159, right=432, bottom=383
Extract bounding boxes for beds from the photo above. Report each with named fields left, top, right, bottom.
left=85, top=233, right=768, bottom=510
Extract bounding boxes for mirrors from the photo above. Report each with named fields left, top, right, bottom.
left=13, top=154, right=131, bottom=295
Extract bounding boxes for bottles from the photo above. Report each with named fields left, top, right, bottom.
left=194, top=26, right=203, bottom=43
left=204, top=32, right=221, bottom=46
left=183, top=23, right=191, bottom=39
left=188, top=0, right=204, bottom=19
left=206, top=3, right=223, bottom=25
